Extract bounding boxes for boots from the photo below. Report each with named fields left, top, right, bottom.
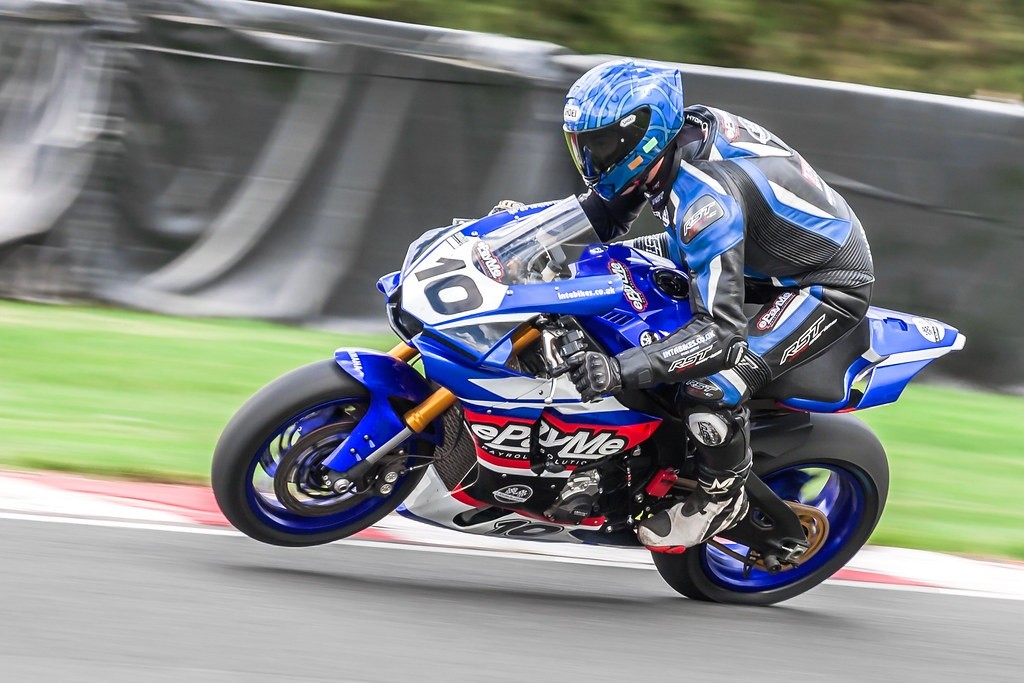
left=636, top=405, right=755, bottom=548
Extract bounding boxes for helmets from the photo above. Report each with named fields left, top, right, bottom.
left=563, top=57, right=686, bottom=202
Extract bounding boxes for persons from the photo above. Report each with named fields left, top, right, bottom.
left=488, top=61, right=876, bottom=552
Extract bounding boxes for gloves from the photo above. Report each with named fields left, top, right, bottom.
left=569, top=351, right=623, bottom=403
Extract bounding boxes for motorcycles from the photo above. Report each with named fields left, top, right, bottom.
left=208, top=189, right=969, bottom=606
